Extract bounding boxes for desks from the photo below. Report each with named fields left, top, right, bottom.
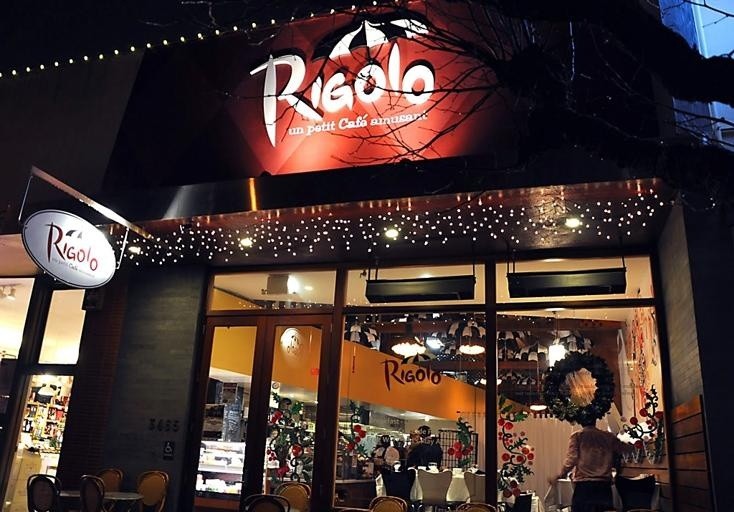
left=556, top=479, right=660, bottom=512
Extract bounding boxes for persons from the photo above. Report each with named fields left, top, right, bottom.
left=546, top=419, right=625, bottom=512
left=370, top=424, right=444, bottom=512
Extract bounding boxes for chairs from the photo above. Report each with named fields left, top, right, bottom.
left=241, top=470, right=500, bottom=512
left=615, top=475, right=655, bottom=512
left=26, top=467, right=169, bottom=512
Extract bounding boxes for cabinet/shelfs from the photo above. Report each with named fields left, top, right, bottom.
left=18, top=402, right=66, bottom=446
left=192, top=441, right=244, bottom=511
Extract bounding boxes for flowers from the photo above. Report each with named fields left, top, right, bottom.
left=541, top=351, right=615, bottom=426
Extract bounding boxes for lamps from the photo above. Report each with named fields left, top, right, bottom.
left=458, top=338, right=485, bottom=355
left=388, top=335, right=425, bottom=357
